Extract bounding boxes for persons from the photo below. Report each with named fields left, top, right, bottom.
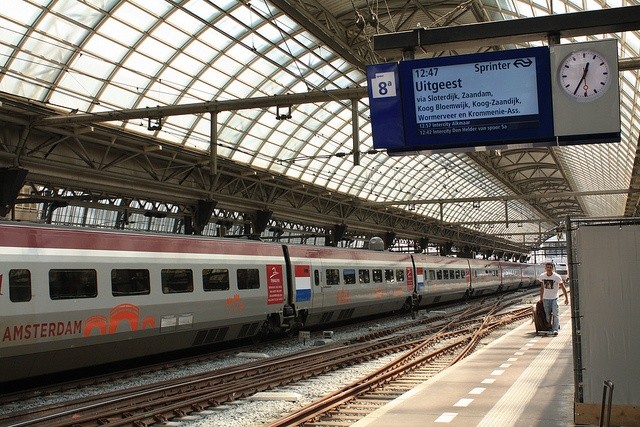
left=539, top=262, right=568, bottom=334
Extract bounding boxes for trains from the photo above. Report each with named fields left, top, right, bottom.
left=0, top=220, right=556, bottom=383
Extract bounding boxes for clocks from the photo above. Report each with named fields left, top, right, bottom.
left=557, top=49, right=612, bottom=103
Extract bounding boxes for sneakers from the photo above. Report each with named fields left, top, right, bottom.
left=553, top=331, right=558, bottom=335
